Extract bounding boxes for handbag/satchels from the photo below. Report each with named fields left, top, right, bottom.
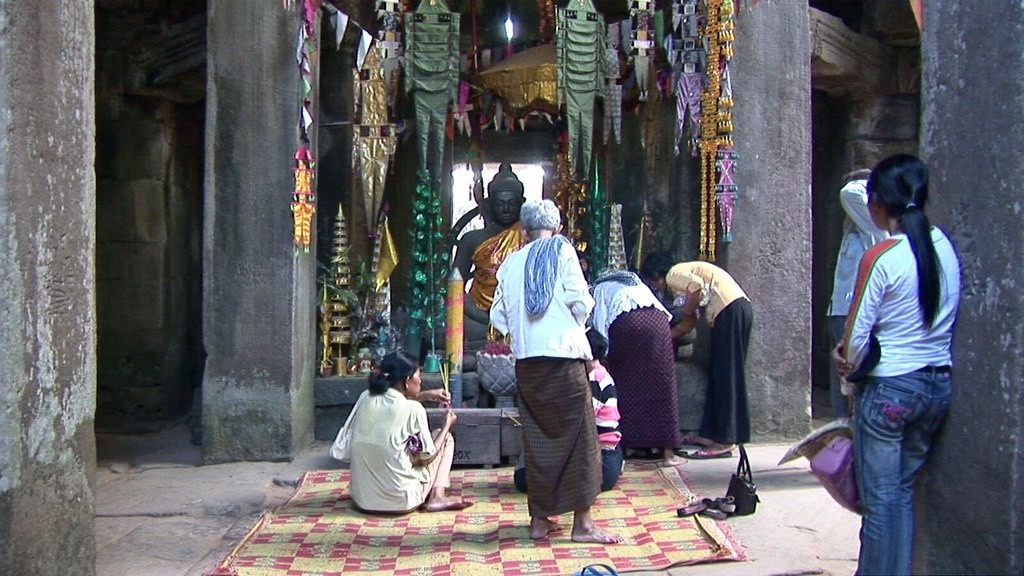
left=791, top=395, right=863, bottom=515
left=329, top=388, right=370, bottom=462
left=725, top=444, right=761, bottom=516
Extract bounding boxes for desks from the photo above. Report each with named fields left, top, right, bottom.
left=501, top=407, right=523, bottom=466
left=426, top=407, right=501, bottom=470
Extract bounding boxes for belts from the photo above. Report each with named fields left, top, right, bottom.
left=916, top=365, right=951, bottom=374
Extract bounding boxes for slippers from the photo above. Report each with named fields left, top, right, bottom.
left=690, top=497, right=738, bottom=520
left=688, top=446, right=733, bottom=459
left=680, top=434, right=707, bottom=448
left=573, top=562, right=618, bottom=575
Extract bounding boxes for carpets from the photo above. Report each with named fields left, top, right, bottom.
left=215, top=463, right=745, bottom=575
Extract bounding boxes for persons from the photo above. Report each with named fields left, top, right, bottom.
left=640, top=251, right=753, bottom=459
left=827, top=153, right=959, bottom=576
left=330, top=352, right=474, bottom=515
left=593, top=270, right=686, bottom=466
left=490, top=200, right=624, bottom=543
left=426, top=161, right=526, bottom=370
left=513, top=326, right=624, bottom=493
